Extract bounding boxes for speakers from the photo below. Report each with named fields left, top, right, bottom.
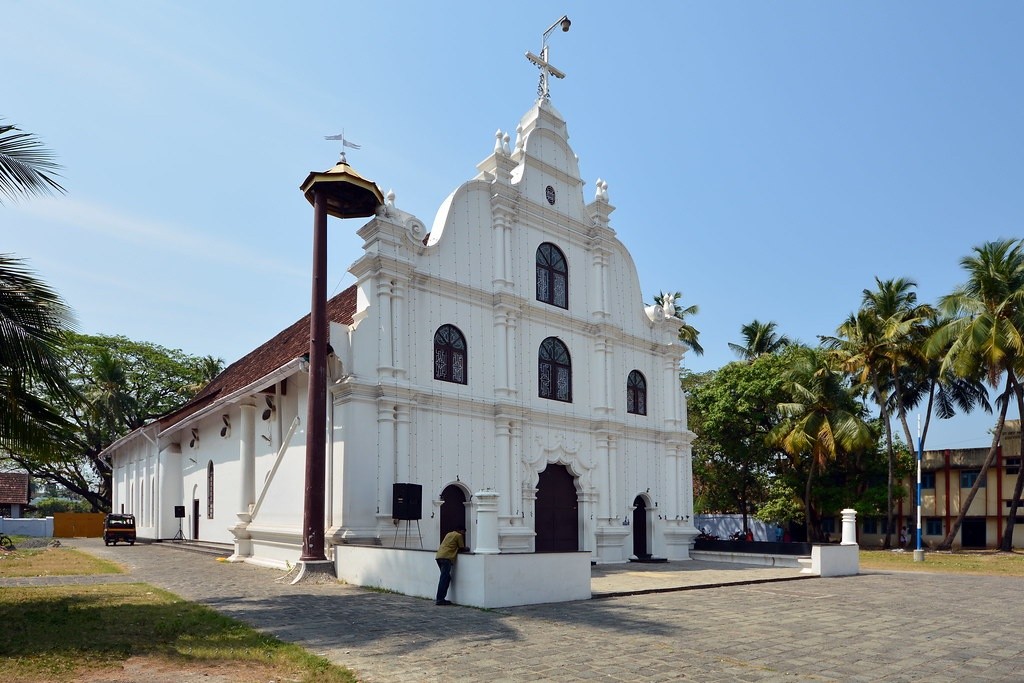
left=175, top=506, right=185, bottom=518
left=392, top=483, right=422, bottom=521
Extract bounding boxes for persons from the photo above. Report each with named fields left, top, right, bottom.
left=775, top=524, right=791, bottom=543
left=435, top=524, right=470, bottom=605
left=746, top=528, right=754, bottom=542
left=900, top=526, right=907, bottom=548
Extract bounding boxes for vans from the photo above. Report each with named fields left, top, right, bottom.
left=102, top=513, right=137, bottom=547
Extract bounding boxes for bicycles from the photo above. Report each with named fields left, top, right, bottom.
left=0, top=532, right=12, bottom=549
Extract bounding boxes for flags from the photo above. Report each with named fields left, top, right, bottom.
left=325, top=134, right=342, bottom=140
left=343, top=139, right=361, bottom=150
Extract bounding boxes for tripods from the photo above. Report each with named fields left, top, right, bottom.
left=172, top=517, right=187, bottom=542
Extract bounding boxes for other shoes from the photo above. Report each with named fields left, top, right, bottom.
left=435, top=600, right=451, bottom=606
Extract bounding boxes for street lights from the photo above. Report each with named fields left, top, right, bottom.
left=299, top=150, right=386, bottom=561
